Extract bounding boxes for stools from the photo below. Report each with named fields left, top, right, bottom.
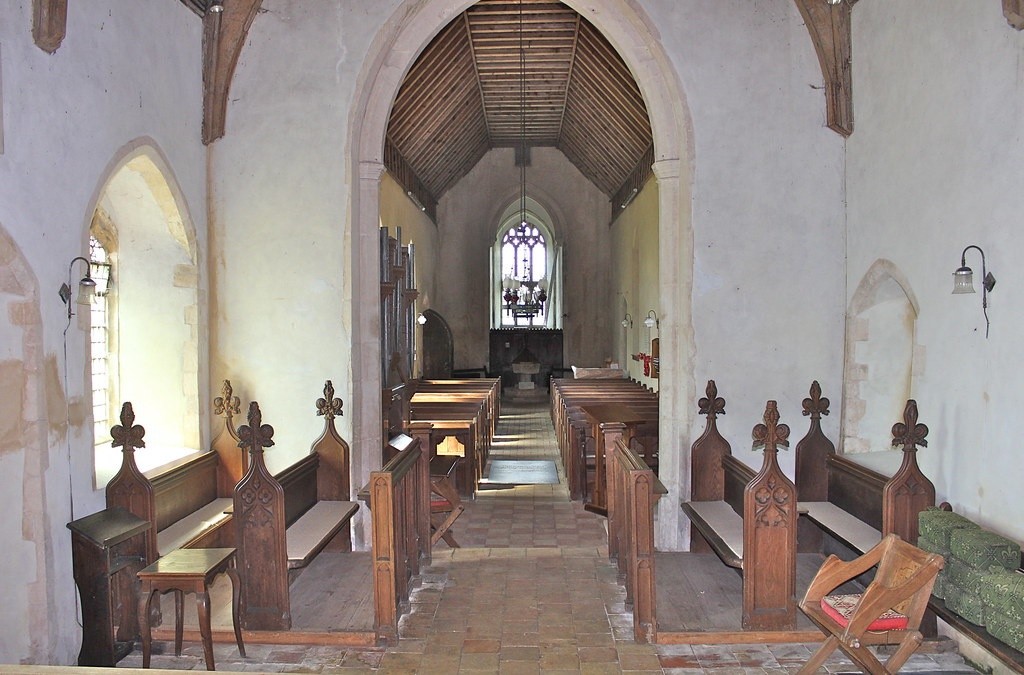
left=430, top=477, right=464, bottom=549
left=138, top=548, right=247, bottom=672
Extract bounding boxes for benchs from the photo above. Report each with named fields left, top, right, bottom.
left=793, top=382, right=940, bottom=639
left=385, top=377, right=501, bottom=500
left=106, top=379, right=248, bottom=627
left=548, top=374, right=659, bottom=500
left=232, top=380, right=359, bottom=633
left=683, top=380, right=800, bottom=632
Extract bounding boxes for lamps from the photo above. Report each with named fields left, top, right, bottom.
left=417, top=313, right=427, bottom=324
left=503, top=0, right=548, bottom=319
left=644, top=309, right=659, bottom=329
left=620, top=314, right=632, bottom=329
left=58, top=256, right=96, bottom=319
left=952, top=245, right=996, bottom=307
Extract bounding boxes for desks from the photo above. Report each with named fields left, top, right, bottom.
left=578, top=403, right=648, bottom=518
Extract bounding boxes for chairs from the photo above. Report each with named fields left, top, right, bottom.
left=798, top=533, right=944, bottom=674
